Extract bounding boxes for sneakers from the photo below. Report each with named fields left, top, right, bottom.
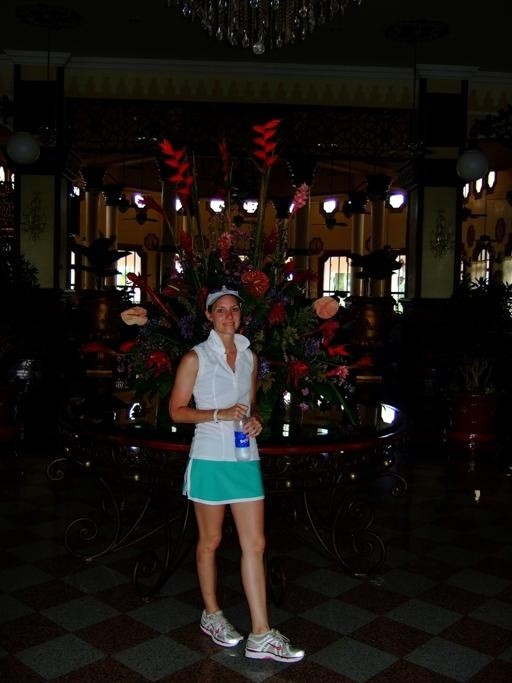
left=200, top=610, right=243, bottom=647
left=245, top=628, right=305, bottom=662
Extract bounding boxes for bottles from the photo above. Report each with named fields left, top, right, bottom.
left=231, top=414, right=251, bottom=464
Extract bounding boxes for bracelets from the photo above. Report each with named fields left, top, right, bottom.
left=213, top=409, right=220, bottom=424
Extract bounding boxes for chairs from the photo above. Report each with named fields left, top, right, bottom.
left=443, top=393, right=499, bottom=472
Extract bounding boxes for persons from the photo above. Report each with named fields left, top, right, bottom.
left=167, top=286, right=305, bottom=665
left=487, top=269, right=508, bottom=296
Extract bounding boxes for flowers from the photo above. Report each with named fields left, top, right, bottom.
left=102, top=116, right=364, bottom=408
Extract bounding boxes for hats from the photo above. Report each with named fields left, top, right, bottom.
left=206, top=285, right=245, bottom=309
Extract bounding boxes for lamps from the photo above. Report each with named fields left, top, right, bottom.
left=163, top=0, right=356, bottom=56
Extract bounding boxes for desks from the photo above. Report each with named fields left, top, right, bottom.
left=43, top=421, right=410, bottom=593
